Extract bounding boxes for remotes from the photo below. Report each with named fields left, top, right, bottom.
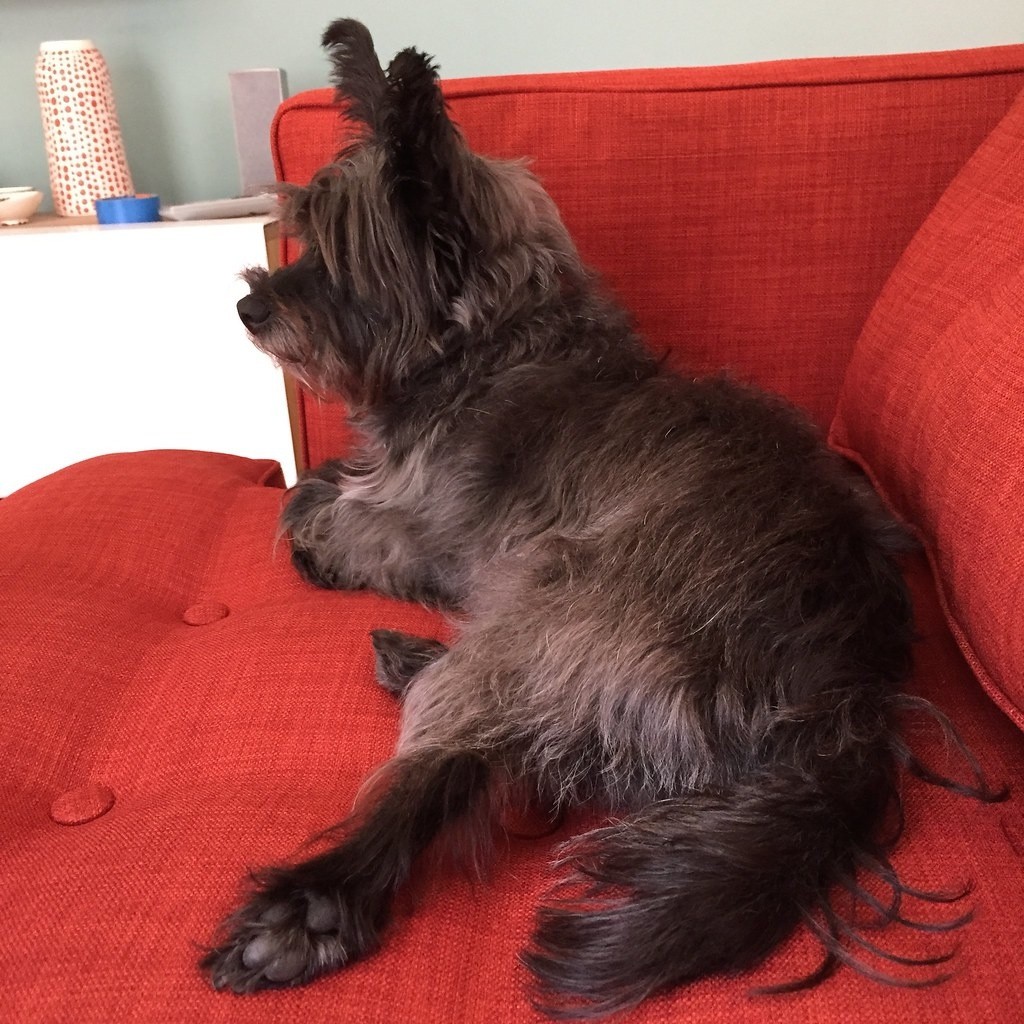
left=156, top=194, right=281, bottom=222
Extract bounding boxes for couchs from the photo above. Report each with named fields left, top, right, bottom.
left=0, top=45, right=1024, bottom=1024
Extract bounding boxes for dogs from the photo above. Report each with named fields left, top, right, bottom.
left=192, top=19, right=1011, bottom=1024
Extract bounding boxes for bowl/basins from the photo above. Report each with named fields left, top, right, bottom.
left=0, top=186, right=36, bottom=193
left=0, top=191, right=44, bottom=225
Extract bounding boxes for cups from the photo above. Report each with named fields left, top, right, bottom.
left=36, top=38, right=161, bottom=222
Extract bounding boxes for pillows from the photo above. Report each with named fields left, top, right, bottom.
left=825, top=87, right=1024, bottom=731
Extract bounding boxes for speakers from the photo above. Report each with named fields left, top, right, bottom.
left=230, top=68, right=289, bottom=198
